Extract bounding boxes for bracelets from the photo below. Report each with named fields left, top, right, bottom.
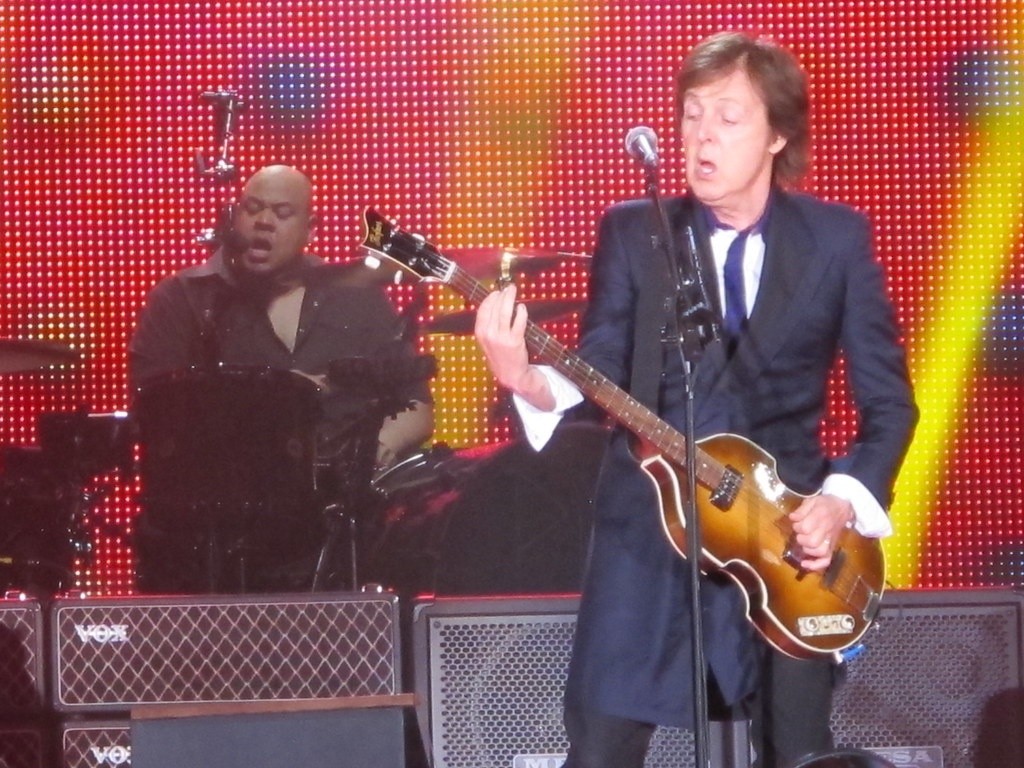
left=846, top=502, right=858, bottom=530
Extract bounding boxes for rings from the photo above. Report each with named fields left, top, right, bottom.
left=825, top=540, right=831, bottom=545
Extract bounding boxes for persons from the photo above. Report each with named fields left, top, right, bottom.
left=125, top=164, right=438, bottom=596
left=474, top=28, right=922, bottom=768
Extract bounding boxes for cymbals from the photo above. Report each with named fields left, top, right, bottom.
left=0, top=333, right=77, bottom=375
left=419, top=295, right=588, bottom=338
left=303, top=243, right=591, bottom=287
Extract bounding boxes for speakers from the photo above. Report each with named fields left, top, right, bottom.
left=0, top=590, right=405, bottom=768
left=829, top=587, right=1024, bottom=768
left=412, top=592, right=751, bottom=768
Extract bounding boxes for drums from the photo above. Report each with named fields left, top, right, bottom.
left=123, top=362, right=329, bottom=534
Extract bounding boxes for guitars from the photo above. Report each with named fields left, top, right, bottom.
left=355, top=201, right=886, bottom=664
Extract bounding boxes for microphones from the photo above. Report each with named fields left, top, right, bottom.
left=625, top=126, right=659, bottom=168
